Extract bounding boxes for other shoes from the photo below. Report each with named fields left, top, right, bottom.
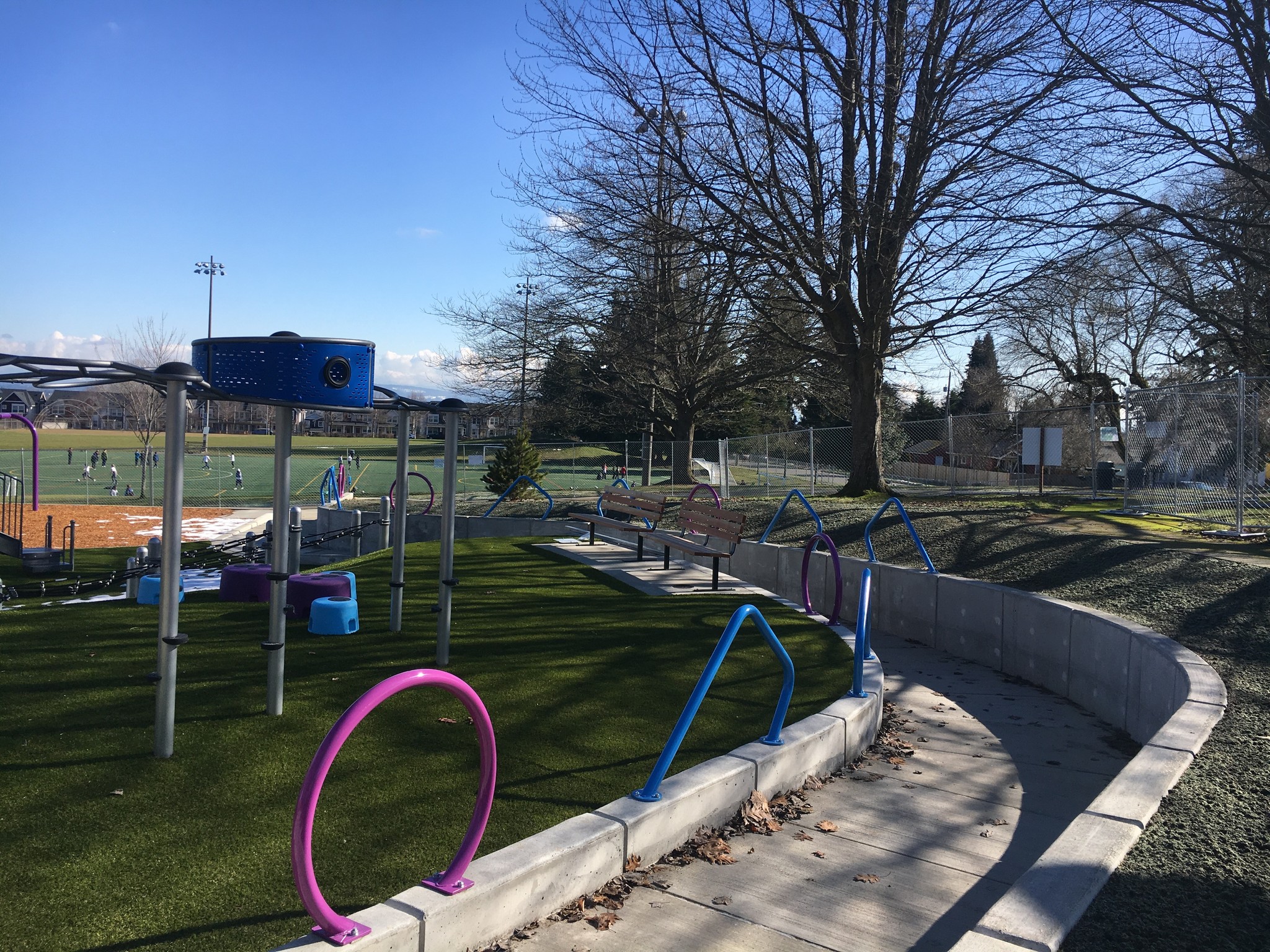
left=115, top=482, right=118, bottom=484
left=157, top=467, right=158, bottom=468
left=93, top=479, right=96, bottom=482
left=154, top=466, right=156, bottom=468
left=202, top=468, right=204, bottom=470
left=104, top=466, right=106, bottom=467
left=111, top=482, right=115, bottom=485
left=615, top=478, right=617, bottom=479
left=241, top=487, right=244, bottom=489
left=358, top=468, right=359, bottom=470
left=357, top=469, right=358, bottom=470
left=324, top=486, right=328, bottom=487
left=233, top=488, right=237, bottom=490
left=348, top=468, right=350, bottom=470
left=604, top=478, right=607, bottom=479
left=83, top=477, right=86, bottom=479
left=136, top=465, right=138, bottom=467
left=232, top=468, right=235, bottom=469
left=102, top=466, right=103, bottom=467
left=209, top=468, right=212, bottom=470
left=622, top=478, right=624, bottom=479
left=94, top=468, right=96, bottom=470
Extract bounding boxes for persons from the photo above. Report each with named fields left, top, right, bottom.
left=90, top=449, right=108, bottom=470
left=139, top=451, right=149, bottom=466
left=68, top=448, right=73, bottom=464
left=135, top=449, right=141, bottom=467
left=124, top=484, right=134, bottom=496
left=338, top=454, right=343, bottom=470
left=597, top=463, right=626, bottom=480
left=153, top=452, right=158, bottom=468
left=202, top=454, right=213, bottom=470
left=348, top=454, right=352, bottom=470
left=227, top=452, right=235, bottom=469
left=631, top=481, right=636, bottom=487
left=351, top=486, right=357, bottom=493
left=337, top=474, right=342, bottom=487
left=110, top=464, right=118, bottom=485
left=234, top=469, right=244, bottom=490
left=110, top=485, right=118, bottom=496
left=739, top=480, right=747, bottom=485
left=355, top=456, right=361, bottom=470
left=346, top=474, right=352, bottom=489
left=82, top=464, right=96, bottom=482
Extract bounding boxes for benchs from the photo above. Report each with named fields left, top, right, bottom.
left=568, top=485, right=667, bottom=563
left=639, top=499, right=746, bottom=592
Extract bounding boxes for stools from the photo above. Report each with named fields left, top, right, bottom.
left=286, top=571, right=359, bottom=635
left=220, top=563, right=269, bottom=603
left=137, top=574, right=185, bottom=606
